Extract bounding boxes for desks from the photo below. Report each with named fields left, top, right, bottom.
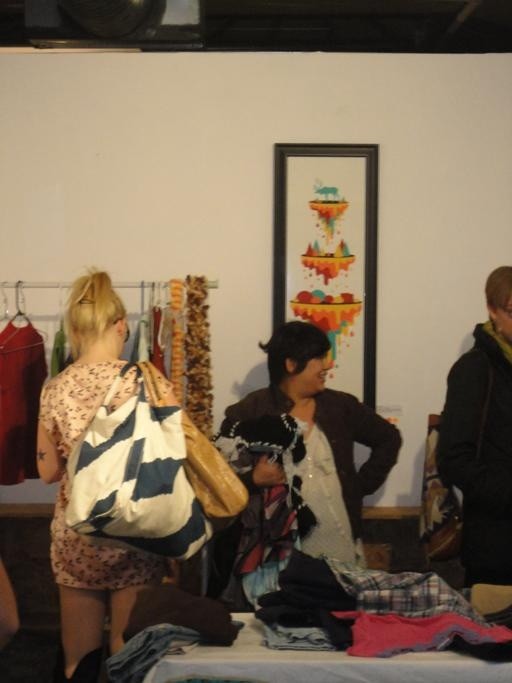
left=362, top=505, right=423, bottom=573
left=140, top=610, right=511, bottom=682
left=2, top=473, right=59, bottom=630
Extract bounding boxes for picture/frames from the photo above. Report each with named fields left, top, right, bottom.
left=271, top=142, right=379, bottom=415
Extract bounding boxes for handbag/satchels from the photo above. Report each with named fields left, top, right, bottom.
left=66, top=362, right=215, bottom=562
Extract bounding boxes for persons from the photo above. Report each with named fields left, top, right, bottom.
left=35, top=271, right=185, bottom=683
left=434, top=267, right=512, bottom=585
left=213, top=321, right=401, bottom=612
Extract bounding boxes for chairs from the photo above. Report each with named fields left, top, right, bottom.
left=470, top=583, right=512, bottom=616
